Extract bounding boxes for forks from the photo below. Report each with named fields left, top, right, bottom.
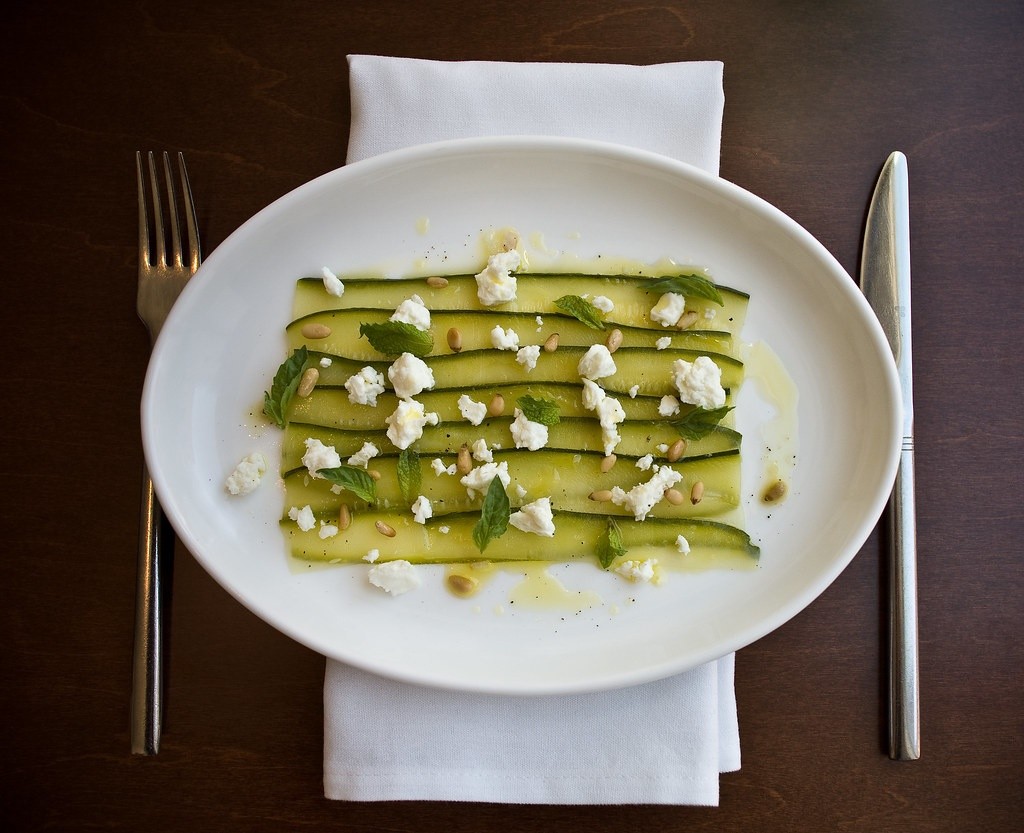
left=132, top=149, right=202, bottom=756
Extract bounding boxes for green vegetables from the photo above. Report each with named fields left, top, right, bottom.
left=516, top=393, right=561, bottom=426
left=637, top=274, right=724, bottom=307
left=473, top=474, right=511, bottom=554
left=669, top=404, right=737, bottom=441
left=593, top=517, right=628, bottom=570
left=263, top=345, right=308, bottom=430
left=552, top=294, right=607, bottom=331
left=358, top=318, right=434, bottom=358
left=314, top=465, right=377, bottom=506
left=398, top=446, right=422, bottom=509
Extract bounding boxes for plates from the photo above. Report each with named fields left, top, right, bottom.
left=139, top=135, right=905, bottom=698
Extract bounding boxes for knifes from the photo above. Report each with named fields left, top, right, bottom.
left=859, top=150, right=922, bottom=761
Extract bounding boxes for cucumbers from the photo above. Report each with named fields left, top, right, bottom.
left=282, top=271, right=762, bottom=564
